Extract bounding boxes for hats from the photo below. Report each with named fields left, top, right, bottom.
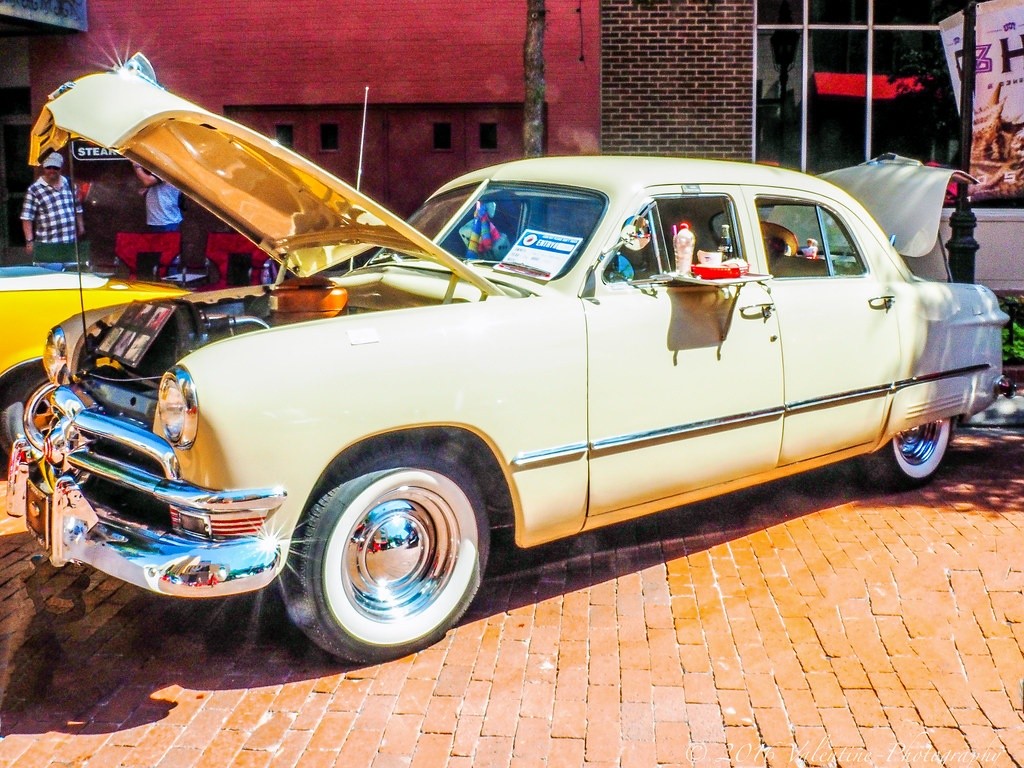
left=43, top=152, right=64, bottom=169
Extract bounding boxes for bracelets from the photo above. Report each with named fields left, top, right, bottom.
left=133, top=164, right=142, bottom=168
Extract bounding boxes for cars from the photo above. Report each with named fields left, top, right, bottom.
left=0, top=259, right=199, bottom=459
left=21, top=70, right=1015, bottom=667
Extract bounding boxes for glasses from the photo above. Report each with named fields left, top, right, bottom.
left=45, top=166, right=60, bottom=171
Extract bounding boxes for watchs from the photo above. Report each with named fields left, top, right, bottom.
left=26, top=239, right=34, bottom=243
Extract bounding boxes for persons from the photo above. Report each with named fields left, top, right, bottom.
left=21, top=154, right=84, bottom=272
left=131, top=156, right=182, bottom=276
